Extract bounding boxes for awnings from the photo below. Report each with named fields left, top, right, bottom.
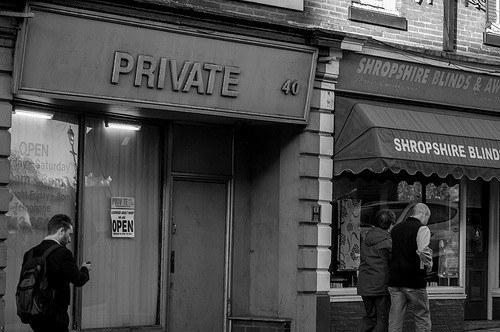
left=334, top=95, right=500, bottom=181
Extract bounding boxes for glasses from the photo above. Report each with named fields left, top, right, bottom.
left=391, top=221, right=396, bottom=225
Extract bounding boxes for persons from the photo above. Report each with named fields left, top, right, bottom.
left=19, top=214, right=92, bottom=332
left=356, top=208, right=397, bottom=332
left=387, top=203, right=433, bottom=332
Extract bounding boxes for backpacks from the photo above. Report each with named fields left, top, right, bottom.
left=15, top=243, right=63, bottom=324
